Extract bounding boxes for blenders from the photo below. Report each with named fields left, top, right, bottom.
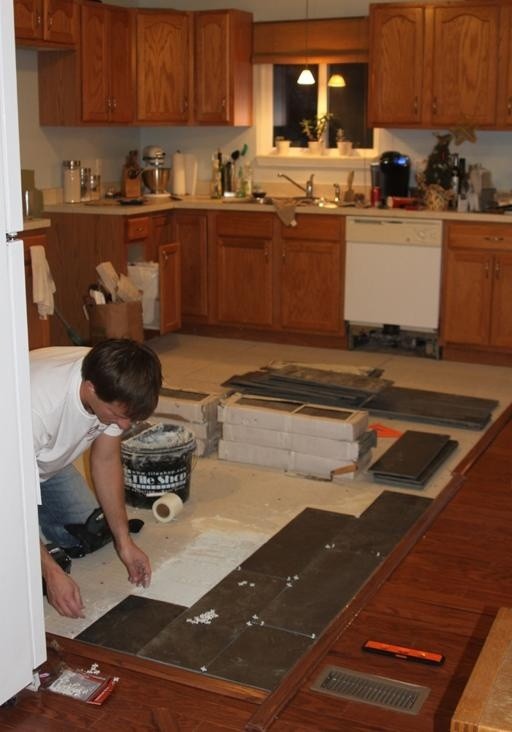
left=142, top=145, right=171, bottom=198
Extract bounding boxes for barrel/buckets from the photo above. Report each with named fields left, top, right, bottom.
left=120, top=422, right=197, bottom=508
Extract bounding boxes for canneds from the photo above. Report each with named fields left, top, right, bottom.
left=371, top=187, right=382, bottom=208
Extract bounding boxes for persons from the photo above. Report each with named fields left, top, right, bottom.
left=28, top=336, right=165, bottom=619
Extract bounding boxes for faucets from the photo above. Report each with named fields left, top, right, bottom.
left=277, top=173, right=314, bottom=197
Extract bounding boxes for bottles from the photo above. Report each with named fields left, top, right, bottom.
left=62, top=160, right=102, bottom=204
left=123, top=150, right=142, bottom=197
left=449, top=153, right=492, bottom=212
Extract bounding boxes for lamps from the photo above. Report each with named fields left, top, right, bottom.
left=296, top=1, right=316, bottom=85
left=328, top=63, right=347, bottom=87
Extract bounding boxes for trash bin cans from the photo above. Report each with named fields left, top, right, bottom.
left=127, top=262, right=161, bottom=330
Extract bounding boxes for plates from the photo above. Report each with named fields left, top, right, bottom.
left=115, top=198, right=149, bottom=205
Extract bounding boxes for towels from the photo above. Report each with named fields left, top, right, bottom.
left=28, top=245, right=56, bottom=320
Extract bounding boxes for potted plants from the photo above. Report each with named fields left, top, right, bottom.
left=299, top=112, right=334, bottom=155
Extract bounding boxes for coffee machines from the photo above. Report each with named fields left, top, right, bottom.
left=370, top=150, right=411, bottom=208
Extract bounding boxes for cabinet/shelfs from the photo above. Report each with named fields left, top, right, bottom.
left=24, top=230, right=51, bottom=351
left=14, top=0, right=255, bottom=128
left=365, top=0, right=512, bottom=130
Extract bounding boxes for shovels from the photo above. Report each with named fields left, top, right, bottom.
left=303, top=464, right=357, bottom=481
